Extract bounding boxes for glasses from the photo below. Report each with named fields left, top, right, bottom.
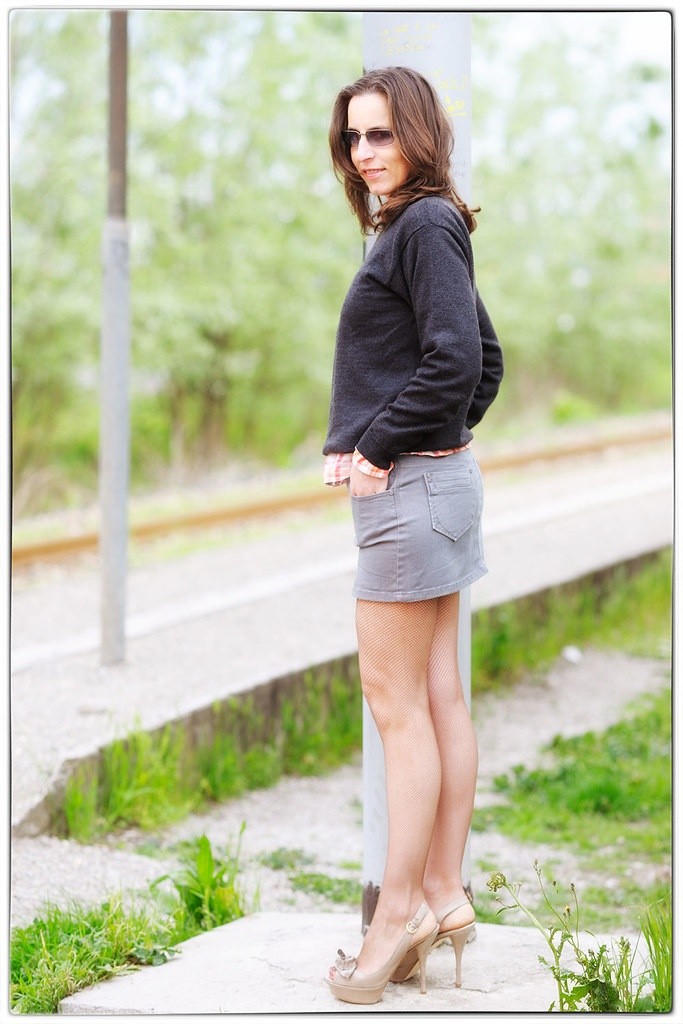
left=341, top=129, right=395, bottom=146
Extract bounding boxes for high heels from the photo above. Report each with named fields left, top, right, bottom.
left=325, top=899, right=438, bottom=1005
left=386, top=897, right=477, bottom=985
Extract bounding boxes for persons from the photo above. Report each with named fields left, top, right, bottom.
left=321, top=65, right=507, bottom=1005
